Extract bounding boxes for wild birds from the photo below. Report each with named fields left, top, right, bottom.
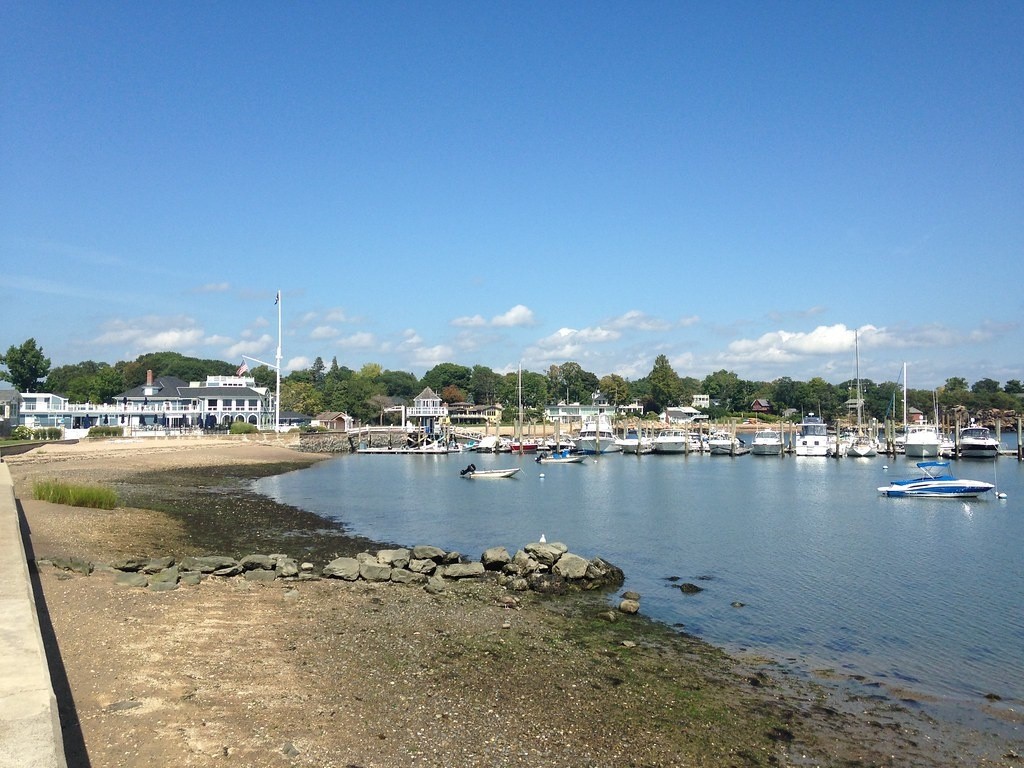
left=538, top=533, right=546, bottom=548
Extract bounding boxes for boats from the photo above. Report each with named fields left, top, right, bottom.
left=511, top=327, right=1000, bottom=458
left=877, top=461, right=996, bottom=498
left=460, top=463, right=520, bottom=479
left=539, top=449, right=587, bottom=466
